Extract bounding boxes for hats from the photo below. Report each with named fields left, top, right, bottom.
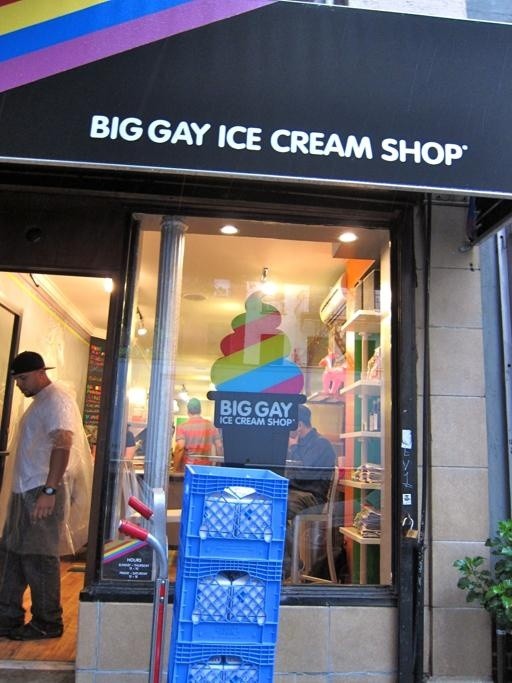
left=187, top=397, right=201, bottom=415
left=10, top=350, right=56, bottom=376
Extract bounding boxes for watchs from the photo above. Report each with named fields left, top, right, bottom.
left=40, top=485, right=58, bottom=496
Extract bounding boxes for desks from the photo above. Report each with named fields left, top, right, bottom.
left=190, top=455, right=302, bottom=469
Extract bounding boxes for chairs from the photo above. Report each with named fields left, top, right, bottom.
left=287, top=466, right=339, bottom=585
left=123, top=467, right=182, bottom=527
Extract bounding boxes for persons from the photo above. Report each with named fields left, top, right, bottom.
left=172, top=396, right=223, bottom=472
left=124, top=422, right=138, bottom=470
left=0, top=349, right=83, bottom=641
left=280, top=402, right=338, bottom=580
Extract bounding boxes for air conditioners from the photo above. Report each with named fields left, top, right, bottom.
left=319, top=272, right=348, bottom=327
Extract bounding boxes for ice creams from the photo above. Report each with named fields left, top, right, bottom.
left=207, top=291, right=306, bottom=478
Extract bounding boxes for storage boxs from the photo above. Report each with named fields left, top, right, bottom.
left=167, top=464, right=289, bottom=682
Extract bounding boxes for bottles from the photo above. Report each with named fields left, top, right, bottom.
left=369, top=398, right=380, bottom=432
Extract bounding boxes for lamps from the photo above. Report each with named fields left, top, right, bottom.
left=138, top=318, right=147, bottom=336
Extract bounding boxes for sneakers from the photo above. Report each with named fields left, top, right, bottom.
left=0, top=620, right=26, bottom=637
left=11, top=618, right=65, bottom=639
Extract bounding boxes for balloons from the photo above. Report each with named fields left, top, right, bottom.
left=318, top=352, right=348, bottom=397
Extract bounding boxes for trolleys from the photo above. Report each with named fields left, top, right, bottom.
left=118, top=495, right=169, bottom=683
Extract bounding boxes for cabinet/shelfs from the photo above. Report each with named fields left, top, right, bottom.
left=338, top=310, right=381, bottom=585
